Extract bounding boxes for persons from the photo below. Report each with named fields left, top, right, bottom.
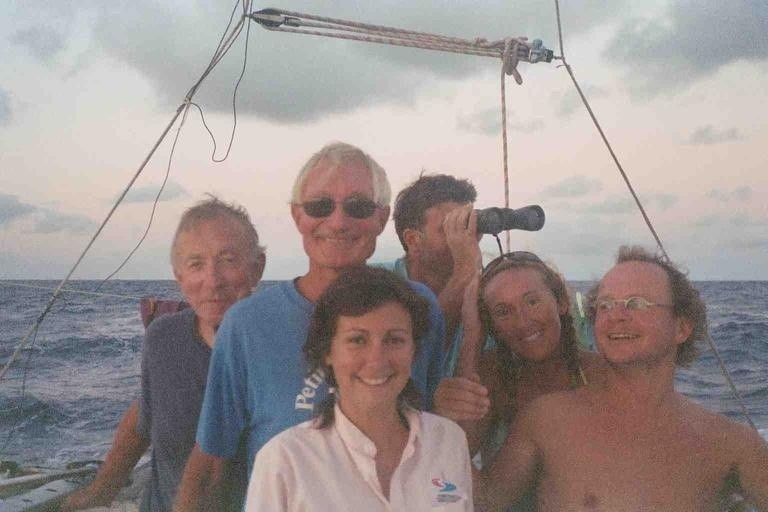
left=167, top=142, right=446, bottom=512
left=386, top=174, right=496, bottom=374
left=455, top=249, right=605, bottom=469
left=243, top=264, right=473, bottom=512
left=58, top=196, right=266, bottom=511
left=433, top=246, right=768, bottom=512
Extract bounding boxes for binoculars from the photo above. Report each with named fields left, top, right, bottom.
left=466, top=205, right=545, bottom=234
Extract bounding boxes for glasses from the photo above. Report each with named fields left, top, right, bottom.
left=587, top=296, right=674, bottom=314
left=296, top=198, right=379, bottom=220
left=478, top=250, right=543, bottom=285
